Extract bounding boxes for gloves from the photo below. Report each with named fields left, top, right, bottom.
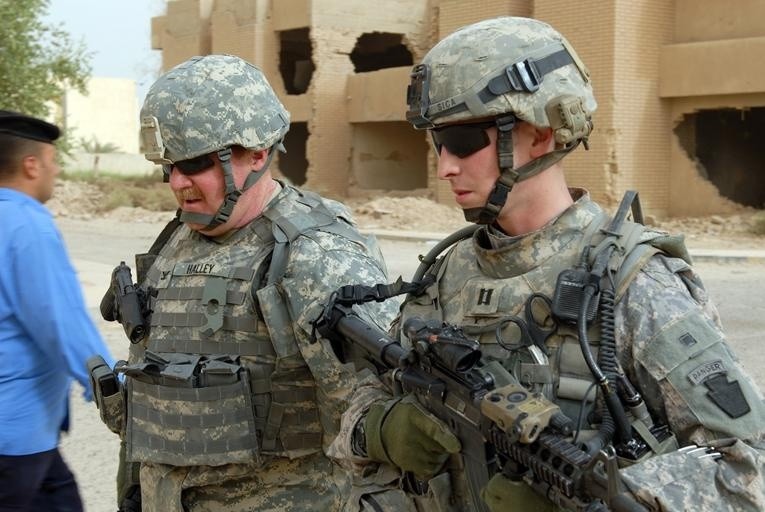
left=482, top=474, right=553, bottom=511
left=365, top=394, right=462, bottom=479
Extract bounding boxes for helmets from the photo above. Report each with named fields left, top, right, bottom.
left=405, top=17, right=597, bottom=151
left=138, top=55, right=290, bottom=165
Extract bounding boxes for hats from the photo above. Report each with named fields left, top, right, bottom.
left=0, top=110, right=61, bottom=143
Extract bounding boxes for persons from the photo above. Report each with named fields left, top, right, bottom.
left=325, top=16, right=765, bottom=512
left=87, top=54, right=401, bottom=512
left=0, top=108, right=126, bottom=512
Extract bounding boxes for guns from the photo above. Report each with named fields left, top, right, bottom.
left=315, top=301, right=651, bottom=511
left=99, top=261, right=145, bottom=343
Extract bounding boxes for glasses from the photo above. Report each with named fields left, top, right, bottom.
left=162, top=155, right=214, bottom=183
left=431, top=118, right=499, bottom=158
